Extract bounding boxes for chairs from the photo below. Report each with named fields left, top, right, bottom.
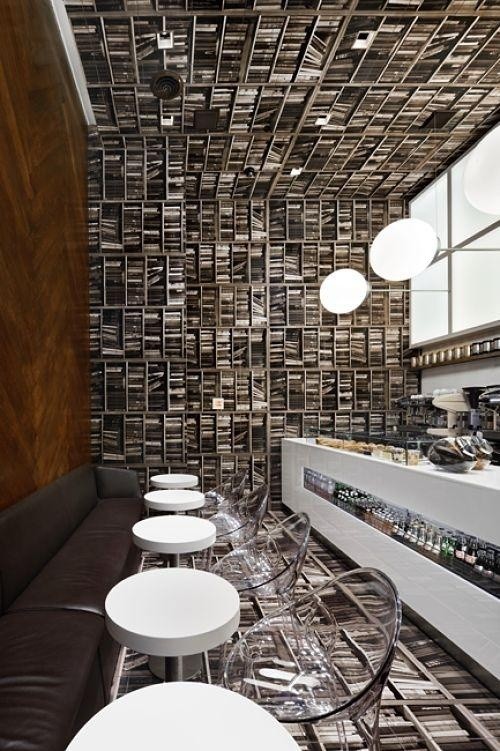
left=197, top=466, right=251, bottom=554
left=208, top=511, right=312, bottom=666
left=223, top=569, right=402, bottom=751
left=200, top=484, right=270, bottom=571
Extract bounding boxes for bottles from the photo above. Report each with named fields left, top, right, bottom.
left=303, top=436, right=500, bottom=587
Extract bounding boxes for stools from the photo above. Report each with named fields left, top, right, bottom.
left=143, top=490, right=207, bottom=515
left=150, top=474, right=199, bottom=489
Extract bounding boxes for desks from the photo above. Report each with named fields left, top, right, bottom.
left=64, top=679, right=300, bottom=750
left=132, top=515, right=216, bottom=566
left=104, top=568, right=240, bottom=677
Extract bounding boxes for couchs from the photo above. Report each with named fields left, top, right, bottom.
left=0, top=465, right=151, bottom=749
